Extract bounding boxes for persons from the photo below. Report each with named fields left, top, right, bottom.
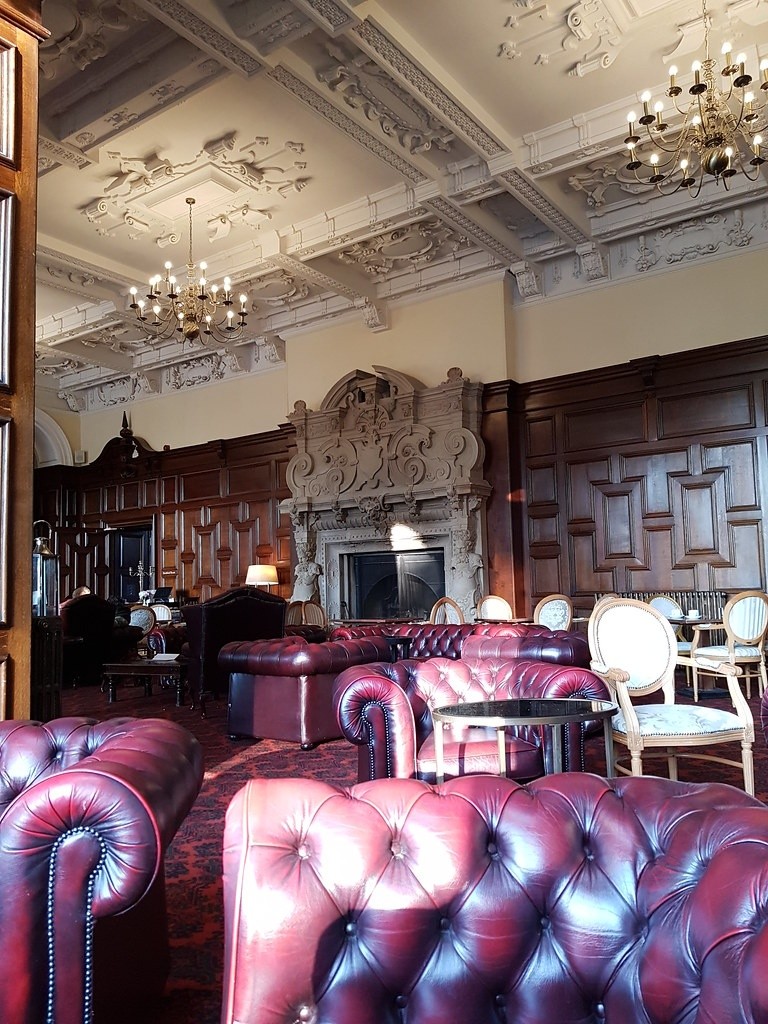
left=60, top=586, right=90, bottom=632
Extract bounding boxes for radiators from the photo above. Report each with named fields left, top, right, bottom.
left=616, top=592, right=727, bottom=644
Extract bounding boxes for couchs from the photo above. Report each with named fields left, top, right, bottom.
left=33, top=587, right=611, bottom=785
left=219, top=773, right=767, bottom=1024
left=0, top=717, right=205, bottom=1022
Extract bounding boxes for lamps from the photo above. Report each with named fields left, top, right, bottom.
left=129, top=195, right=250, bottom=344
left=615, top=1, right=768, bottom=199
left=245, top=565, right=280, bottom=594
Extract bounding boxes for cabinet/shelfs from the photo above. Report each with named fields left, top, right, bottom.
left=0, top=2, right=50, bottom=719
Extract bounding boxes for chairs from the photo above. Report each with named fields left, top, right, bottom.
left=149, top=604, right=172, bottom=625
left=129, top=603, right=157, bottom=638
left=430, top=593, right=766, bottom=802
left=301, top=602, right=329, bottom=629
left=285, top=602, right=303, bottom=624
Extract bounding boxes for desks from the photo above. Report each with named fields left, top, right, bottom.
left=331, top=617, right=424, bottom=627
left=100, top=662, right=195, bottom=708
left=433, top=698, right=619, bottom=779
left=666, top=618, right=732, bottom=699
